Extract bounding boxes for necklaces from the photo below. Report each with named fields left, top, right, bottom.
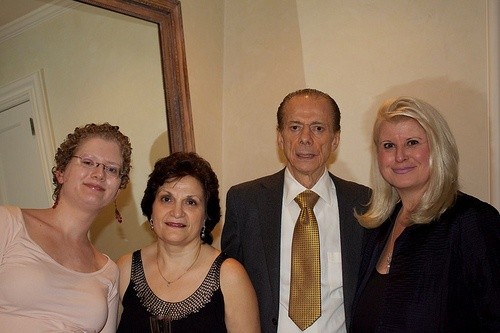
left=156, top=242, right=201, bottom=287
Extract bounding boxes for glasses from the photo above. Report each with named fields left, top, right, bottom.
left=71, top=155, right=122, bottom=178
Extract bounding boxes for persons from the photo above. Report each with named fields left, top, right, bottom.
left=351, top=97, right=500, bottom=333
left=116, top=153, right=259, bottom=333
left=220, top=89, right=373, bottom=333
left=0, top=122, right=133, bottom=333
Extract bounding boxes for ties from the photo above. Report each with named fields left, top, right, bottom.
left=288, top=190, right=321, bottom=332
